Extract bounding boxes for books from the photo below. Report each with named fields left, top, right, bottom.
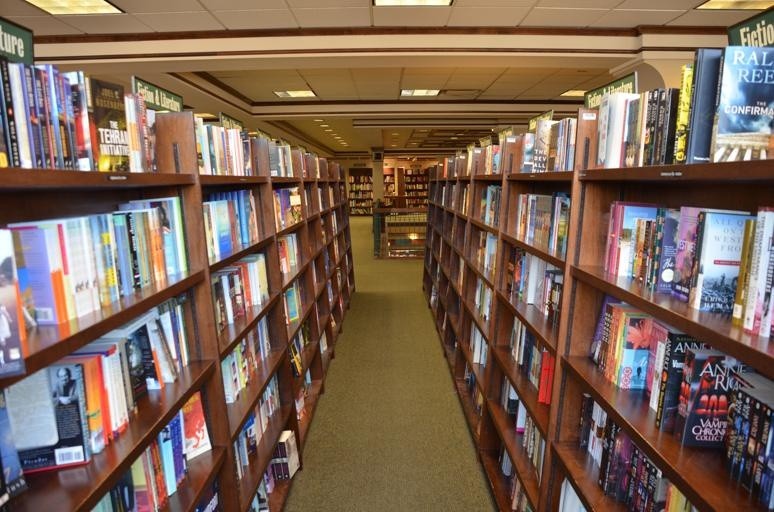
left=1, top=55, right=353, bottom=512
left=429, top=43, right=774, bottom=512
left=357, top=169, right=426, bottom=259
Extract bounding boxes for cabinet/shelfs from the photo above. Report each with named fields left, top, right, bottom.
left=547, top=107, right=773, bottom=512
left=193, top=109, right=355, bottom=512
left=0, top=109, right=239, bottom=512
left=422, top=111, right=583, bottom=512
left=348, top=166, right=428, bottom=216
